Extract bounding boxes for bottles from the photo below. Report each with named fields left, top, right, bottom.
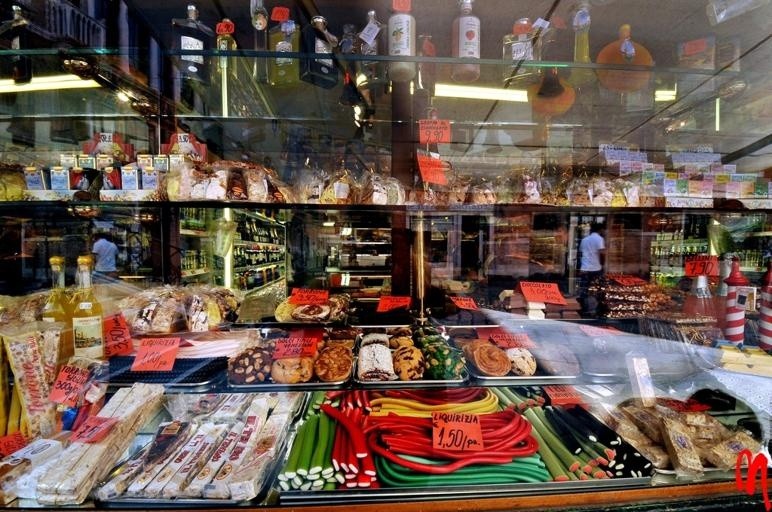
left=501, top=16, right=543, bottom=88
left=450, top=1, right=481, bottom=83
left=650, top=245, right=769, bottom=268
left=170, top=4, right=239, bottom=87
left=40, top=253, right=107, bottom=366
left=264, top=14, right=340, bottom=91
left=232, top=213, right=285, bottom=288
left=681, top=254, right=731, bottom=339
left=355, top=8, right=416, bottom=89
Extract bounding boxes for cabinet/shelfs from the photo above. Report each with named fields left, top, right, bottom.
left=1, top=49, right=770, bottom=511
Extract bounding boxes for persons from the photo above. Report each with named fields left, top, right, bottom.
left=91, top=233, right=119, bottom=280
left=579, top=222, right=606, bottom=274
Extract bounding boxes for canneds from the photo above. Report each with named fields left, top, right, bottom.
left=23, top=153, right=185, bottom=190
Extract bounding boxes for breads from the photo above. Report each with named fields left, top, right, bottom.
left=358, top=334, right=536, bottom=380
left=167, top=159, right=664, bottom=206
left=228, top=337, right=353, bottom=383
left=259, top=327, right=478, bottom=339
left=120, top=287, right=239, bottom=332
